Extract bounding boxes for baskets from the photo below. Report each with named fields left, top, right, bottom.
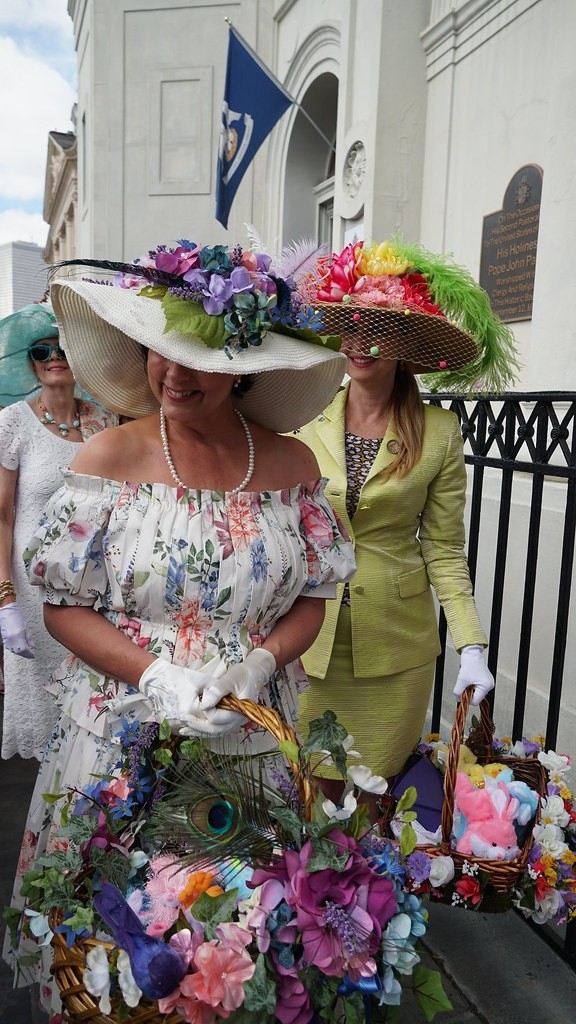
left=48, top=693, right=317, bottom=1024
left=371, top=686, right=565, bottom=914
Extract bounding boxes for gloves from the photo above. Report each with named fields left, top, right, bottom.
left=0, top=602, right=37, bottom=659
left=453, top=644, right=494, bottom=705
left=179, top=648, right=277, bottom=740
left=139, top=657, right=221, bottom=736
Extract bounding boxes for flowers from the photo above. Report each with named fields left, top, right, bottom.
left=109, top=237, right=324, bottom=355
left=0, top=718, right=434, bottom=1024
left=407, top=727, right=576, bottom=925
left=304, top=241, right=448, bottom=318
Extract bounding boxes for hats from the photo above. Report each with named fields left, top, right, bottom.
left=295, top=241, right=525, bottom=400
left=49, top=240, right=348, bottom=436
left=0, top=290, right=105, bottom=409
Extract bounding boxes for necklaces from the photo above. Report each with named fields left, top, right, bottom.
left=159, top=405, right=254, bottom=493
left=38, top=396, right=80, bottom=436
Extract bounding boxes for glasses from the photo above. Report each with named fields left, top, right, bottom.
left=27, top=344, right=66, bottom=362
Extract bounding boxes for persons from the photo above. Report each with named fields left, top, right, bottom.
left=292, top=241, right=494, bottom=831
left=0, top=241, right=357, bottom=1024
left=0, top=304, right=121, bottom=764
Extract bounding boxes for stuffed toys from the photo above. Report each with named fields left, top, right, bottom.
left=432, top=744, right=538, bottom=861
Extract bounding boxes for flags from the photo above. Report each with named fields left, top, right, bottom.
left=215, top=27, right=293, bottom=230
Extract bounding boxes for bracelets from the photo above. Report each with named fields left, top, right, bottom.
left=0, top=579, right=16, bottom=600
